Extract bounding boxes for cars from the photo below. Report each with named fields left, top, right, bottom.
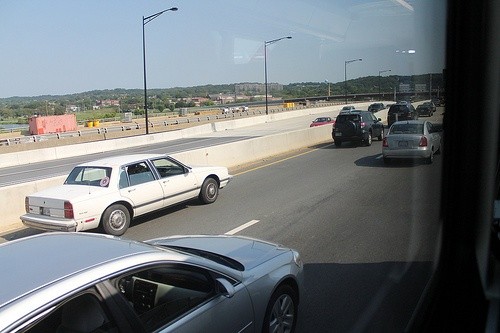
left=382, top=120, right=442, bottom=164
left=21, top=154, right=234, bottom=236
left=340, top=96, right=446, bottom=127
left=0, top=231, right=305, bottom=332
left=310, top=117, right=335, bottom=127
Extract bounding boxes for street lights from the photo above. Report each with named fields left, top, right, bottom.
left=345, top=59, right=363, bottom=104
left=264, top=37, right=292, bottom=115
left=378, top=69, right=391, bottom=101
left=141, top=7, right=179, bottom=134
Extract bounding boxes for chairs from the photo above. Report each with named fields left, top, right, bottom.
left=60, top=295, right=107, bottom=333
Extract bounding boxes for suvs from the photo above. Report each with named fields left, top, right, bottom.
left=332, top=110, right=383, bottom=147
